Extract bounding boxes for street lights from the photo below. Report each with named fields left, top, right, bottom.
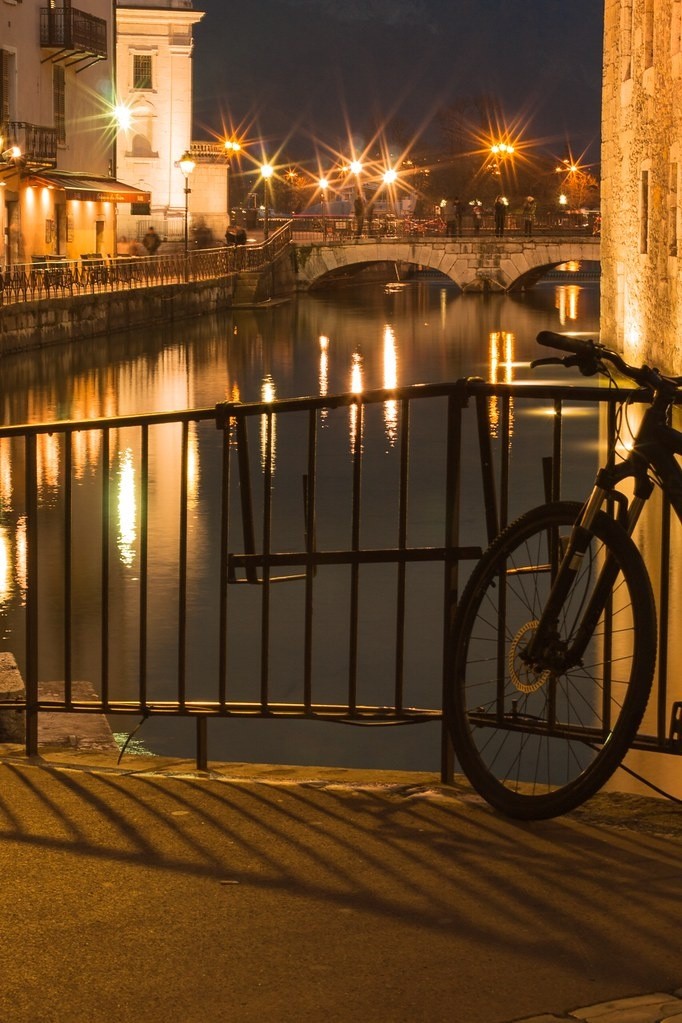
left=319, top=178, right=328, bottom=241
left=178, top=150, right=196, bottom=284
left=383, top=169, right=397, bottom=235
left=261, top=164, right=274, bottom=240
left=351, top=161, right=362, bottom=229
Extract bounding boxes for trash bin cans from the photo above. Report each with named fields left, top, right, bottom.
left=31, top=254, right=67, bottom=284
left=80, top=253, right=104, bottom=277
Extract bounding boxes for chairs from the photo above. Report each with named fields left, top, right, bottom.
left=0, top=254, right=139, bottom=303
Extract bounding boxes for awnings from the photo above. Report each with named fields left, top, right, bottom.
left=18, top=173, right=151, bottom=204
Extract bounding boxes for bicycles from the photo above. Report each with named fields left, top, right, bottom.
left=442, top=329, right=681, bottom=825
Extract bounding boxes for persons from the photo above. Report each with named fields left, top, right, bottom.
left=116, top=227, right=161, bottom=282
left=444, top=195, right=537, bottom=238
left=225, top=224, right=247, bottom=270
